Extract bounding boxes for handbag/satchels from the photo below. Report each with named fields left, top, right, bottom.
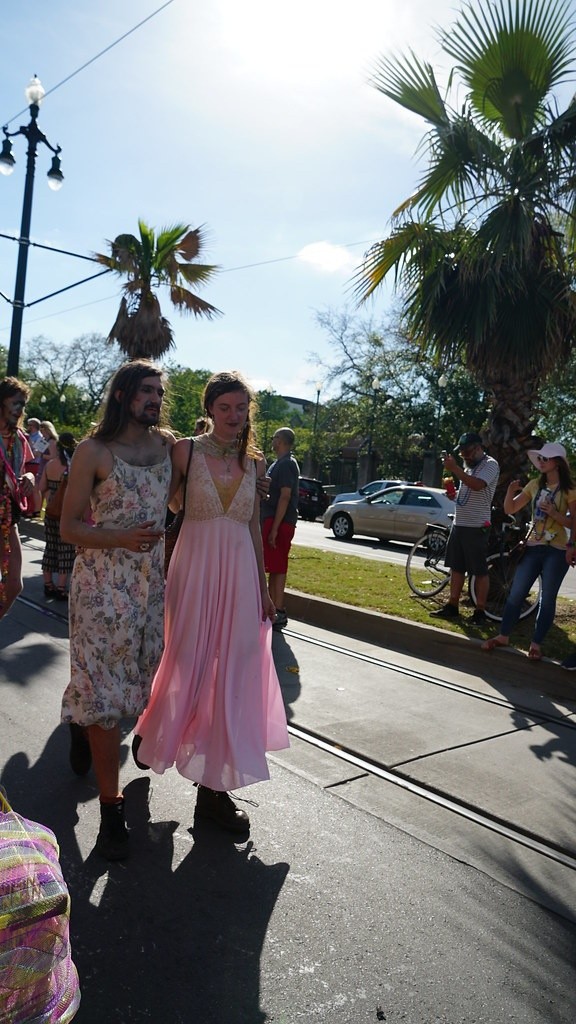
left=15, top=481, right=36, bottom=514
left=509, top=540, right=528, bottom=563
left=164, top=510, right=185, bottom=573
left=45, top=467, right=68, bottom=522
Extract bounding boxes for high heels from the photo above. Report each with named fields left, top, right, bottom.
left=44, top=581, right=58, bottom=597
left=56, top=585, right=70, bottom=602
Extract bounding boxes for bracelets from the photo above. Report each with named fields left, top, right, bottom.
left=41, top=453, right=43, bottom=458
left=565, top=541, right=576, bottom=547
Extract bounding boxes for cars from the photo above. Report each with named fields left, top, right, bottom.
left=323, top=480, right=459, bottom=543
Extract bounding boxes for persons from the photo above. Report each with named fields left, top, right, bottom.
left=61, top=364, right=272, bottom=861
left=428, top=433, right=501, bottom=628
left=194, top=417, right=211, bottom=436
left=75, top=372, right=278, bottom=832
left=259, top=427, right=300, bottom=625
left=0, top=376, right=214, bottom=621
left=0, top=784, right=82, bottom=1024
left=480, top=443, right=576, bottom=661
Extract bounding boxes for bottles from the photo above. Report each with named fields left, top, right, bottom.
left=440, top=450, right=452, bottom=488
left=535, top=487, right=551, bottom=521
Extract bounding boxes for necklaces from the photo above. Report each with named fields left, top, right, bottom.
left=532, top=482, right=561, bottom=541
left=0, top=431, right=15, bottom=456
left=456, top=454, right=487, bottom=506
left=223, top=457, right=234, bottom=473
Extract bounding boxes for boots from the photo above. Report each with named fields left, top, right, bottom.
left=68, top=722, right=92, bottom=775
left=96, top=800, right=130, bottom=861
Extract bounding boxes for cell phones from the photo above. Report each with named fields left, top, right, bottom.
left=441, top=450, right=448, bottom=459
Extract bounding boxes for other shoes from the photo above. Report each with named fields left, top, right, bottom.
left=132, top=734, right=151, bottom=770
left=271, top=608, right=288, bottom=624
left=194, top=781, right=250, bottom=829
left=562, top=653, right=576, bottom=671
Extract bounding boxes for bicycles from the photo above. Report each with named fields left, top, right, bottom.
left=406, top=505, right=542, bottom=622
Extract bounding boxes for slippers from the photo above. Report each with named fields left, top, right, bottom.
left=528, top=648, right=542, bottom=661
left=480, top=639, right=510, bottom=649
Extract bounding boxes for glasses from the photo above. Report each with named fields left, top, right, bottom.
left=271, top=436, right=279, bottom=440
left=460, top=445, right=479, bottom=458
left=537, top=456, right=561, bottom=462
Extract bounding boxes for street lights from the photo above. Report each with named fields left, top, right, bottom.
left=59, top=393, right=67, bottom=423
left=368, top=377, right=381, bottom=454
left=41, top=395, right=47, bottom=421
left=431, top=373, right=448, bottom=460
left=82, top=392, right=88, bottom=413
left=313, top=381, right=324, bottom=434
left=262, top=386, right=273, bottom=456
left=0, top=74, right=65, bottom=380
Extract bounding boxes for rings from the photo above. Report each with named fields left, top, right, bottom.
left=140, top=543, right=150, bottom=551
left=266, top=494, right=271, bottom=498
left=274, top=614, right=277, bottom=616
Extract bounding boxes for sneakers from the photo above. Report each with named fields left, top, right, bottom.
left=428, top=604, right=460, bottom=620
left=464, top=610, right=487, bottom=625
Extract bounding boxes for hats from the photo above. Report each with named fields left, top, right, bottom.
left=528, top=442, right=568, bottom=472
left=454, top=431, right=482, bottom=451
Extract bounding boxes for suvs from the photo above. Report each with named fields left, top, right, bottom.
left=298, top=476, right=330, bottom=521
